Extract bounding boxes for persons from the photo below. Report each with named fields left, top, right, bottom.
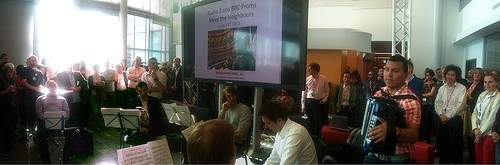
left=258, top=54, right=500, bottom=165
left=218, top=86, right=251, bottom=155
left=186, top=119, right=236, bottom=165
left=0, top=53, right=182, bottom=165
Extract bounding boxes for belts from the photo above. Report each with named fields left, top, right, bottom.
left=341, top=105, right=349, bottom=109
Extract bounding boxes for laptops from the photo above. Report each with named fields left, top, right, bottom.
left=328, top=114, right=352, bottom=131
